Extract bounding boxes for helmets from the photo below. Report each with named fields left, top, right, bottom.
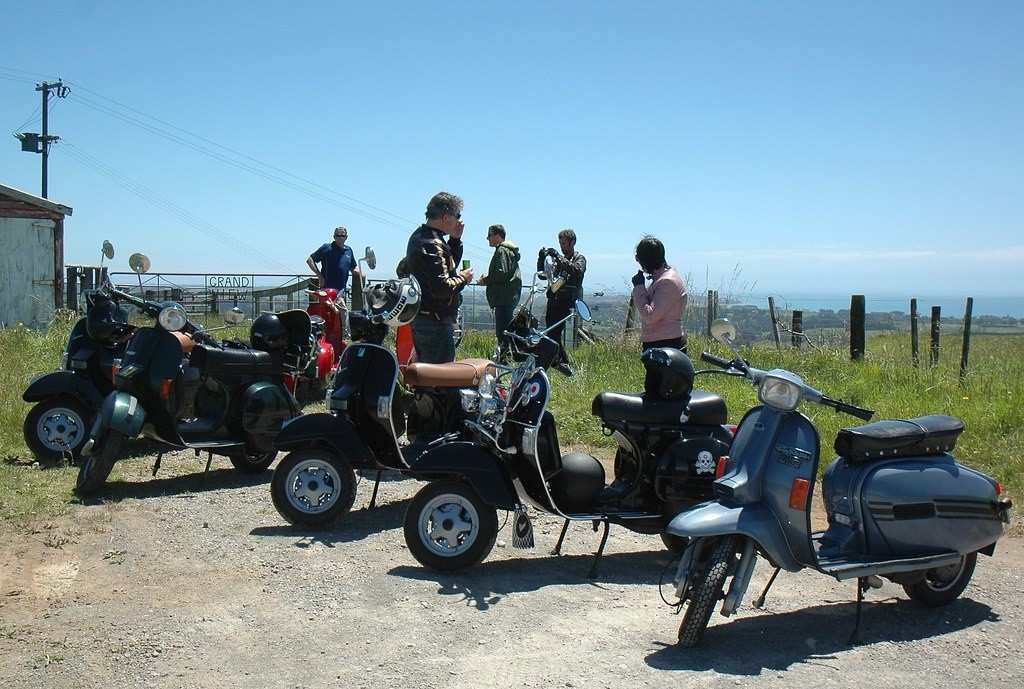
left=85, top=301, right=139, bottom=349
left=249, top=313, right=290, bottom=357
left=366, top=274, right=422, bottom=326
left=560, top=453, right=606, bottom=501
left=639, top=347, right=694, bottom=401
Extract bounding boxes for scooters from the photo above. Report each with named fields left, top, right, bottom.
left=402, top=250, right=744, bottom=579
left=268, top=243, right=509, bottom=526
left=22, top=236, right=350, bottom=499
left=661, top=318, right=1016, bottom=648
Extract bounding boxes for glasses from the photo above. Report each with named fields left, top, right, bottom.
left=488, top=233, right=496, bottom=237
left=452, top=213, right=462, bottom=219
left=335, top=235, right=347, bottom=237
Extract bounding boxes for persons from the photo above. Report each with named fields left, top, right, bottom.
left=476, top=225, right=522, bottom=361
left=537, top=230, right=586, bottom=344
left=632, top=235, right=687, bottom=355
left=395, top=191, right=475, bottom=365
left=307, top=227, right=366, bottom=303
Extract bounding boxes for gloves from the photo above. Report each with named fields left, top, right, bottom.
left=632, top=270, right=645, bottom=286
left=548, top=248, right=557, bottom=257
left=539, top=247, right=548, bottom=259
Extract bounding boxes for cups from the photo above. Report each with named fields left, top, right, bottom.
left=463, top=259, right=470, bottom=271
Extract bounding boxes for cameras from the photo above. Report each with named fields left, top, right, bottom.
left=544, top=250, right=550, bottom=256
